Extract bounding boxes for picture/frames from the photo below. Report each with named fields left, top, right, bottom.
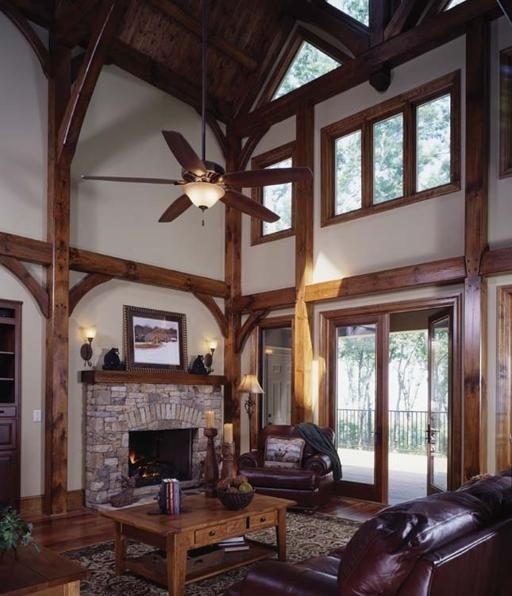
left=122, top=304, right=188, bottom=374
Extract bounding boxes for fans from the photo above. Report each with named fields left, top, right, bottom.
left=80, top=0, right=313, bottom=221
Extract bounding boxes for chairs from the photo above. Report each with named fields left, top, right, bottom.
left=236, top=424, right=334, bottom=512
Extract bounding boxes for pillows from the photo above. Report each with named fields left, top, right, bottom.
left=263, top=435, right=305, bottom=469
left=337, top=471, right=512, bottom=596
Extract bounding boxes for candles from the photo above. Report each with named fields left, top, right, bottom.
left=205, top=409, right=214, bottom=430
left=223, top=422, right=234, bottom=444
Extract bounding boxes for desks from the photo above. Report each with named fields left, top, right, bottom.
left=0, top=534, right=88, bottom=596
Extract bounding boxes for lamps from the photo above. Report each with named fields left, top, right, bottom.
left=202, top=340, right=216, bottom=374
left=182, top=179, right=225, bottom=227
left=80, top=325, right=96, bottom=367
left=236, top=374, right=265, bottom=452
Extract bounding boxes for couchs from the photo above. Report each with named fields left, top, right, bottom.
left=226, top=516, right=512, bottom=596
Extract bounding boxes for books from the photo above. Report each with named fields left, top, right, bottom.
left=216, top=536, right=250, bottom=553
left=161, top=478, right=180, bottom=514
